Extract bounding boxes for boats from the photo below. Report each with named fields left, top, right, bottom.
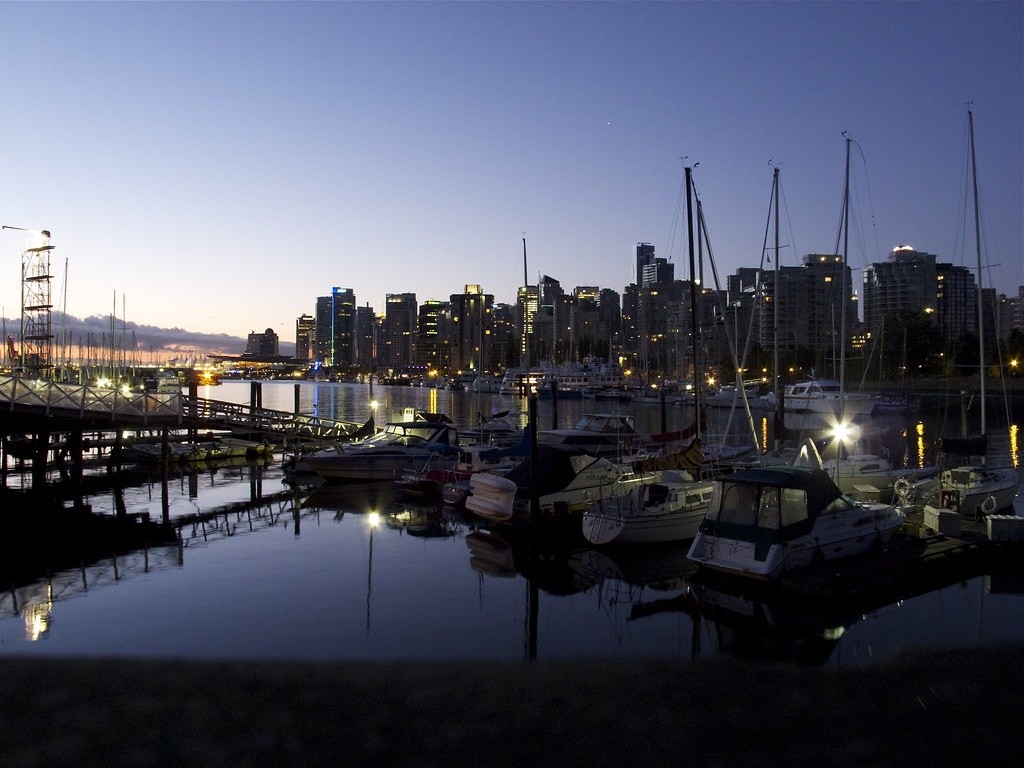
left=132, top=437, right=276, bottom=464
left=298, top=111, right=1023, bottom=671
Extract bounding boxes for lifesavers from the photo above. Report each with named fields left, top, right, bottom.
left=894, top=478, right=911, bottom=498
left=636, top=448, right=648, bottom=457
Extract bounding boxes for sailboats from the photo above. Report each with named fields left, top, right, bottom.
left=2, top=317, right=223, bottom=391
